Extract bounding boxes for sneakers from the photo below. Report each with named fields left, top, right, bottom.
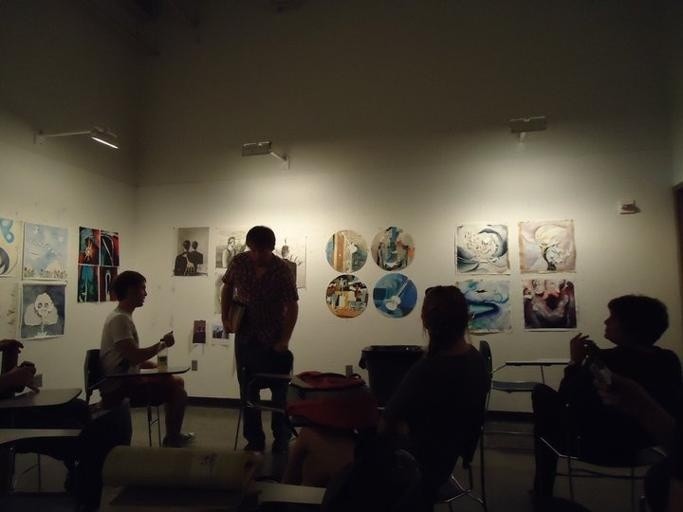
left=163, top=432, right=196, bottom=447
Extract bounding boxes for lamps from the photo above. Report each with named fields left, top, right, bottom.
left=507, top=113, right=548, bottom=141
left=242, top=140, right=290, bottom=169
left=34, top=126, right=120, bottom=149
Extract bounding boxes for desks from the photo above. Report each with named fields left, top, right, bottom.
left=0, top=387, right=83, bottom=492
left=89, top=364, right=190, bottom=447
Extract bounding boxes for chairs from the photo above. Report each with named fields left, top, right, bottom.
left=84, top=348, right=161, bottom=448
left=539, top=436, right=661, bottom=512
left=482, top=357, right=571, bottom=436
left=233, top=366, right=299, bottom=451
left=450, top=340, right=492, bottom=509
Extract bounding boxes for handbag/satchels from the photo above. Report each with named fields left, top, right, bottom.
left=285, top=373, right=381, bottom=430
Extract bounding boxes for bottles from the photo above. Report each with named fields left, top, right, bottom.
left=588, top=362, right=617, bottom=387
left=345, top=365, right=353, bottom=378
left=157, top=339, right=168, bottom=370
left=12, top=361, right=34, bottom=392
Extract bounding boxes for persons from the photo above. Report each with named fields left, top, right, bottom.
left=340, top=282, right=349, bottom=291
left=188, top=241, right=203, bottom=274
left=326, top=282, right=338, bottom=309
left=220, top=226, right=299, bottom=452
left=354, top=284, right=361, bottom=302
left=174, top=241, right=196, bottom=276
left=322, top=286, right=482, bottom=512
left=0, top=340, right=34, bottom=398
left=528, top=295, right=683, bottom=512
left=523, top=280, right=576, bottom=328
left=99, top=270, right=194, bottom=447
left=222, top=237, right=236, bottom=267
